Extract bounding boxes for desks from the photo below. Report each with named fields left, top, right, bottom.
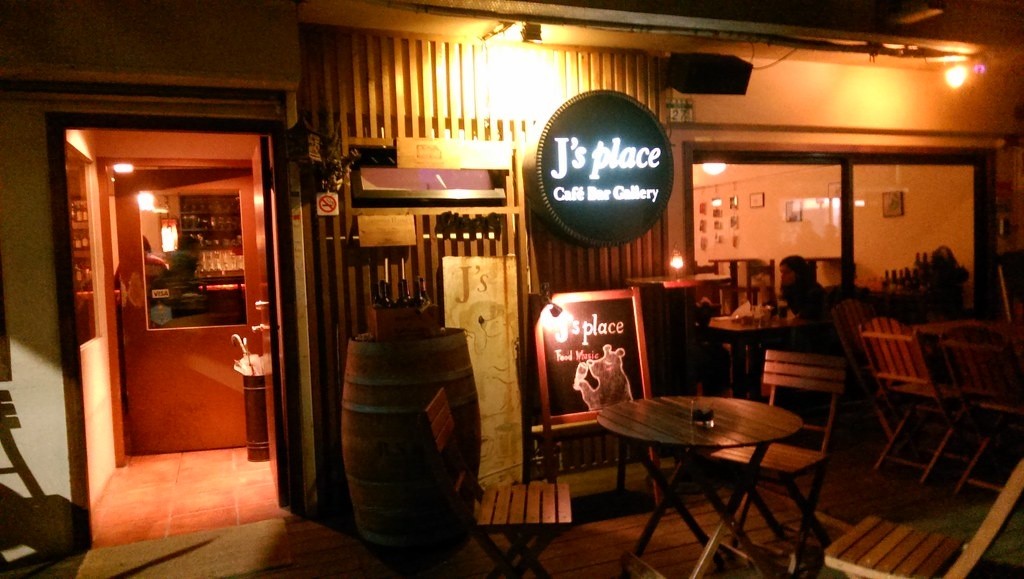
left=705, top=304, right=836, bottom=400
left=596, top=394, right=800, bottom=579
left=148, top=268, right=246, bottom=291
left=894, top=321, right=1024, bottom=473
left=623, top=268, right=730, bottom=316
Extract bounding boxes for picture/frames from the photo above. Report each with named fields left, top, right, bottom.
left=784, top=201, right=803, bottom=222
left=881, top=189, right=905, bottom=217
left=749, top=192, right=765, bottom=208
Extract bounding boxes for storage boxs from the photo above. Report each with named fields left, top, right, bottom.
left=365, top=303, right=440, bottom=340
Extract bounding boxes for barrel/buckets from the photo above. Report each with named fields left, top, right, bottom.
left=341, top=327, right=481, bottom=546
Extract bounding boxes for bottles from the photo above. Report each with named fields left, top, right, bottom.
left=372, top=276, right=431, bottom=310
left=883, top=252, right=928, bottom=293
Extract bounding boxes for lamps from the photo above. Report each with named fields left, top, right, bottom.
left=538, top=279, right=563, bottom=318
left=520, top=22, right=544, bottom=43
left=670, top=241, right=684, bottom=278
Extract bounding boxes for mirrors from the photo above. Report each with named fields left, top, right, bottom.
left=139, top=190, right=248, bottom=331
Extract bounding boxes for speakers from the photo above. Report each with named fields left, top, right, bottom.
left=667, top=53, right=753, bottom=95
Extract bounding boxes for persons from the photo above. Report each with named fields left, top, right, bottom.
left=780, top=256, right=825, bottom=320
left=113, top=235, right=167, bottom=288
left=695, top=297, right=724, bottom=396
left=922, top=246, right=969, bottom=321
left=171, top=237, right=201, bottom=277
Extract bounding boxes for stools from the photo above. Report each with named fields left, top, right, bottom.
left=422, top=387, right=578, bottom=579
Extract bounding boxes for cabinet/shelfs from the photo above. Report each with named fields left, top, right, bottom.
left=178, top=195, right=242, bottom=250
left=71, top=191, right=120, bottom=308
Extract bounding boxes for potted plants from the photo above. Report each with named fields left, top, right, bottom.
left=169, top=249, right=199, bottom=279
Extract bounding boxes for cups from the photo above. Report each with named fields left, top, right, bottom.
left=691, top=396, right=715, bottom=427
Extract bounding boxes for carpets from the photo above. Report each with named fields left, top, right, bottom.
left=0, top=517, right=293, bottom=579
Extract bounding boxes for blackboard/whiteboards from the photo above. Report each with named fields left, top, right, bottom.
left=535, top=286, right=652, bottom=425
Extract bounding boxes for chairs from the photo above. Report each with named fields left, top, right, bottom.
left=699, top=255, right=1024, bottom=579
